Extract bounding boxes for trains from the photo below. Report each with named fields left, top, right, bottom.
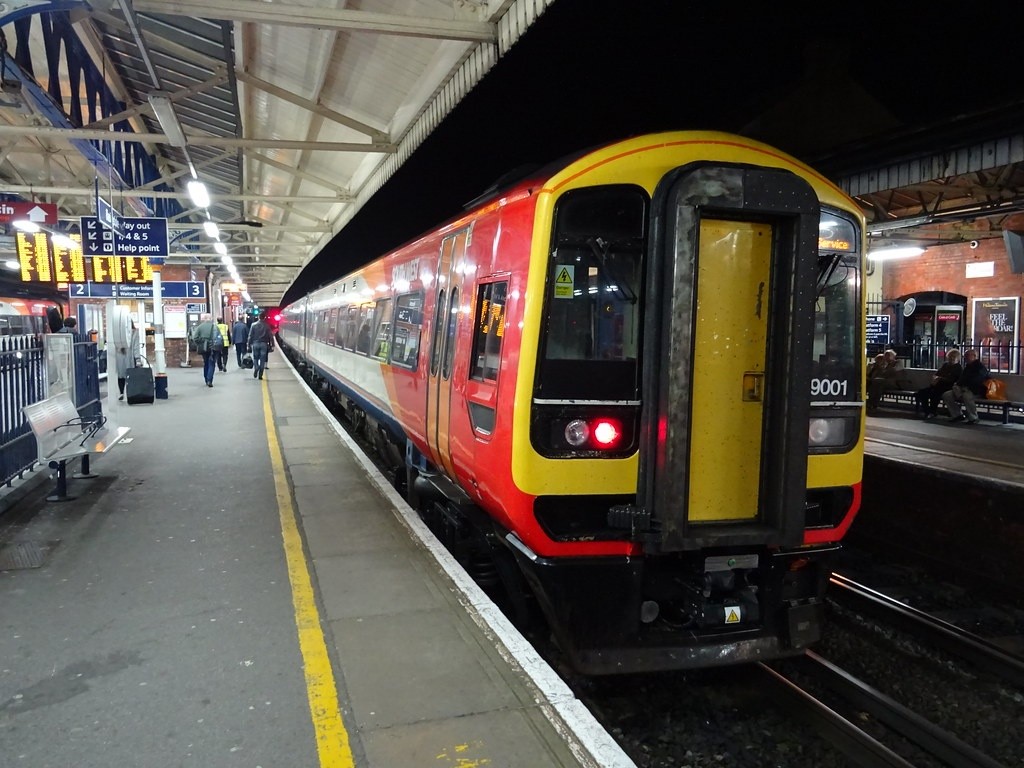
left=273, top=128, right=878, bottom=688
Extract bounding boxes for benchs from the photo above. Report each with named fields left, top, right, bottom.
left=882, top=390, right=920, bottom=416
left=19, top=391, right=132, bottom=501
left=976, top=399, right=1023, bottom=424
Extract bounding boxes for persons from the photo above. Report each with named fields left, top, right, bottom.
left=232, top=313, right=274, bottom=380
left=943, top=350, right=989, bottom=425
left=867, top=350, right=904, bottom=415
left=917, top=349, right=963, bottom=419
left=116, top=321, right=141, bottom=400
left=603, top=314, right=623, bottom=359
left=337, top=315, right=369, bottom=352
left=194, top=315, right=224, bottom=387
left=58, top=317, right=78, bottom=334
left=216, top=316, right=232, bottom=372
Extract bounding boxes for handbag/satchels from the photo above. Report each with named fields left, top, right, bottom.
left=196, top=338, right=213, bottom=354
left=213, top=326, right=224, bottom=351
left=267, top=344, right=274, bottom=352
left=895, top=379, right=920, bottom=391
left=983, top=379, right=1007, bottom=401
left=241, top=350, right=254, bottom=369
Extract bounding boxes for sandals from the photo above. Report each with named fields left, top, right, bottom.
left=924, top=410, right=931, bottom=419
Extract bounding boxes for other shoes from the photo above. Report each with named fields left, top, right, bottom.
left=254, top=370, right=257, bottom=377
left=259, top=374, right=262, bottom=379
left=926, top=411, right=936, bottom=419
left=949, top=415, right=965, bottom=423
left=118, top=394, right=124, bottom=400
left=219, top=366, right=223, bottom=371
left=223, top=368, right=226, bottom=372
left=264, top=367, right=269, bottom=369
left=965, top=419, right=980, bottom=425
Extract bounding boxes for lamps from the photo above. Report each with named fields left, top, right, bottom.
left=148, top=90, right=188, bottom=147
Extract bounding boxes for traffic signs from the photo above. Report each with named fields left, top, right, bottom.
left=96, top=197, right=128, bottom=238
left=79, top=215, right=171, bottom=259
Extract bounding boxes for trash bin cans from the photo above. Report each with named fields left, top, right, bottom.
left=155, top=373, right=169, bottom=399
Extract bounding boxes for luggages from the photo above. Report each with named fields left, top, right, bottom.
left=126, top=365, right=154, bottom=405
